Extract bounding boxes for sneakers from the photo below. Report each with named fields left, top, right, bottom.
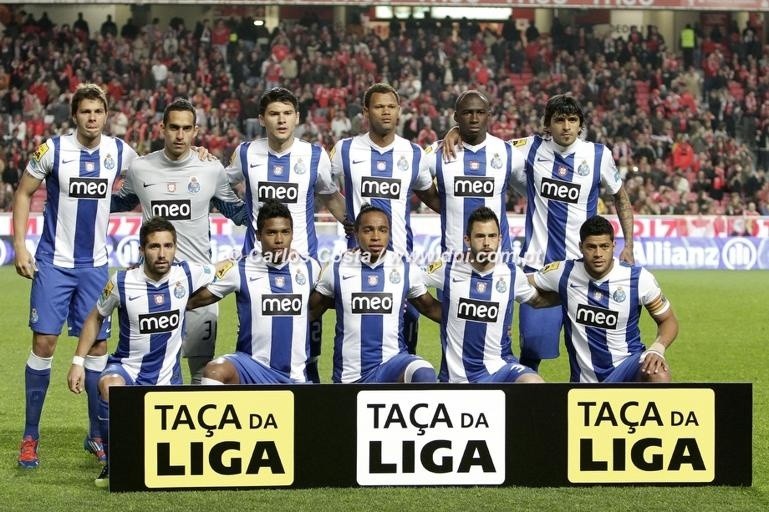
left=18, top=437, right=40, bottom=468
left=94, top=462, right=110, bottom=488
left=83, top=435, right=108, bottom=464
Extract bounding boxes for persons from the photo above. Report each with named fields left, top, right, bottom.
left=1, top=8, right=769, bottom=218
left=14, top=84, right=681, bottom=490
